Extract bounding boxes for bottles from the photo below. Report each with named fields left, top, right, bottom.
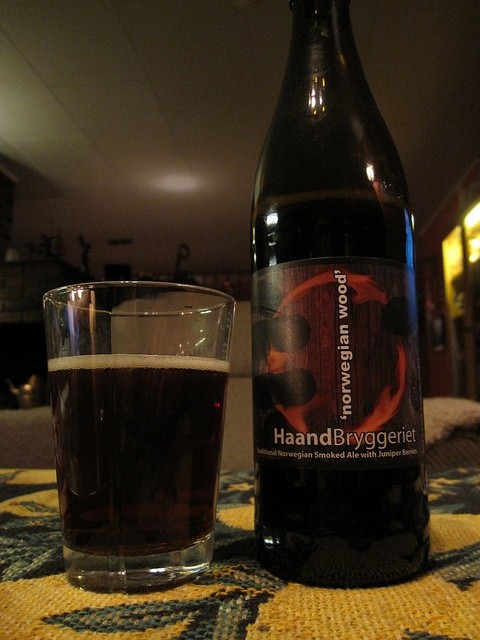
left=251, top=0, right=429, bottom=587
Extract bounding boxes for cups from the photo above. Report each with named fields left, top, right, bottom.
left=44, top=280, right=234, bottom=590
